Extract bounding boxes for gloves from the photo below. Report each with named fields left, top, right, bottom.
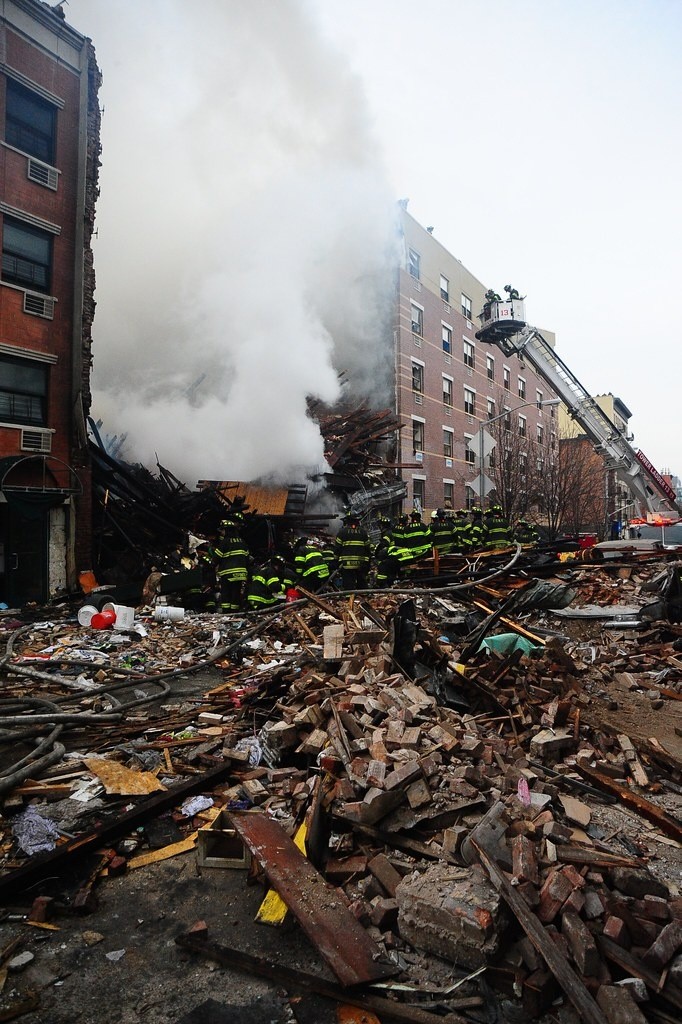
left=363, top=560, right=370, bottom=574
left=332, top=557, right=339, bottom=572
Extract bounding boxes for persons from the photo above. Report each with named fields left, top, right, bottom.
left=483, top=285, right=519, bottom=316
left=177, top=510, right=372, bottom=615
left=375, top=503, right=540, bottom=588
left=621, top=524, right=643, bottom=539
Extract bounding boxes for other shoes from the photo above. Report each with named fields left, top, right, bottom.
left=514, top=778, right=531, bottom=809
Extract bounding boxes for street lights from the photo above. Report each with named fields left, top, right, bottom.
left=479, top=398, right=561, bottom=512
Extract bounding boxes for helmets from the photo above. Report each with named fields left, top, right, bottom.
left=469, top=507, right=482, bottom=514
left=347, top=510, right=361, bottom=521
left=517, top=519, right=527, bottom=526
left=435, top=508, right=446, bottom=516
left=430, top=510, right=437, bottom=518
left=491, top=505, right=502, bottom=516
left=218, top=520, right=234, bottom=530
left=409, top=510, right=421, bottom=518
left=233, top=513, right=244, bottom=522
left=293, top=539, right=299, bottom=546
left=529, top=525, right=535, bottom=530
left=398, top=513, right=407, bottom=522
left=377, top=516, right=390, bottom=522
left=271, top=556, right=286, bottom=568
left=483, top=509, right=492, bottom=519
left=454, top=510, right=468, bottom=516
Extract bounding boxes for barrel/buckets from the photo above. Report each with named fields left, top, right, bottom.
left=156, top=606, right=184, bottom=622
left=103, top=602, right=127, bottom=624
left=91, top=609, right=116, bottom=630
left=79, top=605, right=98, bottom=627
left=114, top=607, right=135, bottom=631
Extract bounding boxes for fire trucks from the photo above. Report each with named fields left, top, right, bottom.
left=473, top=298, right=682, bottom=546
